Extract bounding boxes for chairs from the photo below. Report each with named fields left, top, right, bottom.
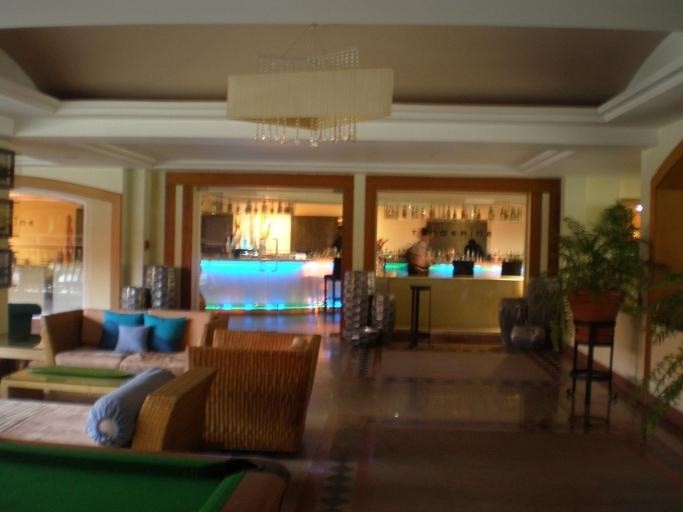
left=188, top=328, right=321, bottom=453
left=324, top=258, right=342, bottom=311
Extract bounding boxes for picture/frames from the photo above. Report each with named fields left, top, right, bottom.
left=0, top=148, right=15, bottom=288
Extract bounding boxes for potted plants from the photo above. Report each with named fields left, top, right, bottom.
left=511, top=198, right=669, bottom=357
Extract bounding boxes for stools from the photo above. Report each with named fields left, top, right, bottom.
left=410, top=285, right=431, bottom=350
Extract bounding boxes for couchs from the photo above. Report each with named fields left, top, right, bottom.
left=39, top=308, right=229, bottom=376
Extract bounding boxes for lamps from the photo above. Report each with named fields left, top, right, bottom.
left=227, top=24, right=394, bottom=148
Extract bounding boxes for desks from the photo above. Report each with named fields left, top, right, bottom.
left=0, top=438, right=290, bottom=512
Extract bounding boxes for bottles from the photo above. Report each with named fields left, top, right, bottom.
left=376, top=246, right=524, bottom=262
left=209, top=199, right=296, bottom=216
left=382, top=203, right=521, bottom=221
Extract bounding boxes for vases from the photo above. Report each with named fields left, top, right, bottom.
left=499, top=298, right=528, bottom=348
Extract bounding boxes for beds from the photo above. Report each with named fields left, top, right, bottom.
left=0, top=367, right=218, bottom=451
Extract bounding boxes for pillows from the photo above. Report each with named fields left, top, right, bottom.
left=85, top=368, right=175, bottom=447
left=97, top=309, right=187, bottom=353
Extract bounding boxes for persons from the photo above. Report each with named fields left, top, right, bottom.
left=464, top=239, right=484, bottom=257
left=328, top=227, right=342, bottom=276
left=406, top=227, right=435, bottom=275
